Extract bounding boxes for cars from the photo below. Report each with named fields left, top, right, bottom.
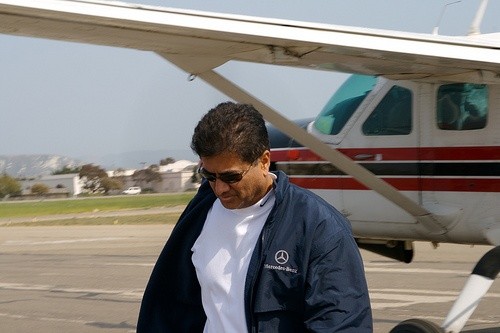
left=122, top=187, right=141, bottom=195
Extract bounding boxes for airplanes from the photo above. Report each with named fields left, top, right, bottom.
left=0, top=0, right=500, bottom=333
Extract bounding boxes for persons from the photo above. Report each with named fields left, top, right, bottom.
left=137, top=102, right=374, bottom=333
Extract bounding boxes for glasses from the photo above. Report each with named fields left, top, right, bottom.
left=199, top=162, right=256, bottom=183
